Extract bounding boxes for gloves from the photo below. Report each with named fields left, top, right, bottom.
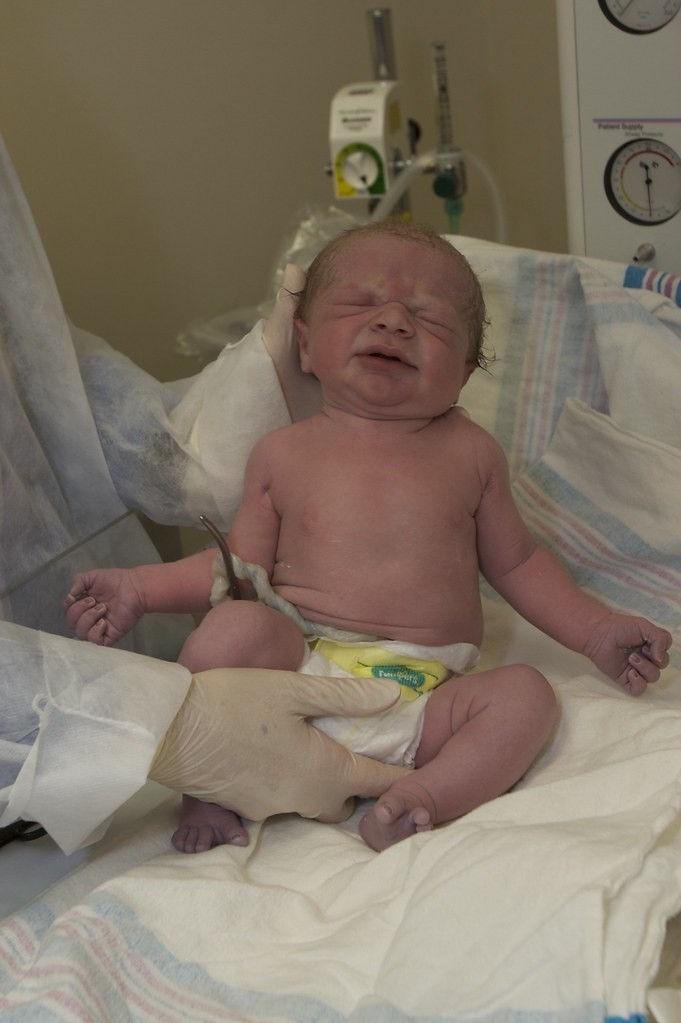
left=148, top=670, right=421, bottom=823
left=264, top=263, right=322, bottom=387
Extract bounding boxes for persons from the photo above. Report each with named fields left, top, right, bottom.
left=0, top=142, right=419, bottom=854
left=64, top=219, right=674, bottom=854
